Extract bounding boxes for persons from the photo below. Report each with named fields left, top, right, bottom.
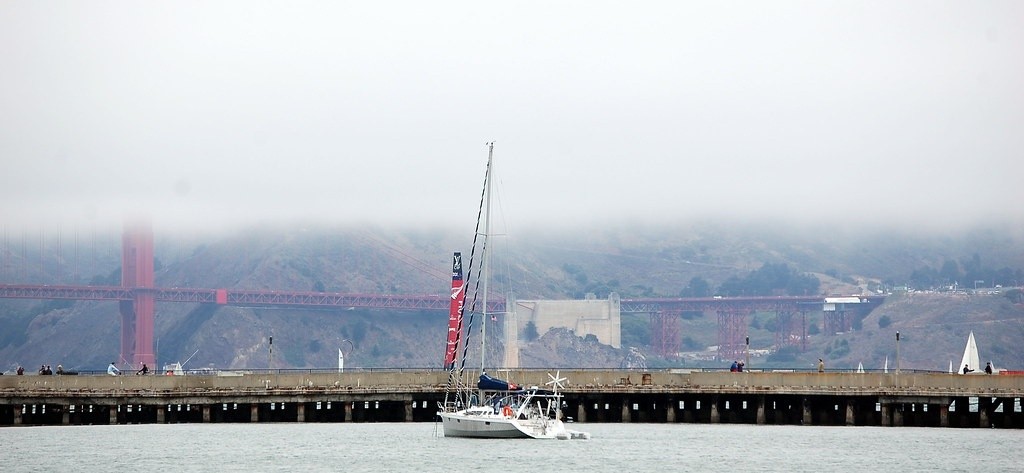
left=56, top=364, right=64, bottom=376
left=137, top=364, right=148, bottom=375
left=985, top=362, right=992, bottom=375
left=17, top=367, right=24, bottom=375
left=107, top=361, right=120, bottom=376
left=818, top=358, right=825, bottom=373
left=39, top=365, right=52, bottom=376
left=737, top=362, right=745, bottom=372
left=730, top=361, right=738, bottom=372
left=963, top=364, right=975, bottom=375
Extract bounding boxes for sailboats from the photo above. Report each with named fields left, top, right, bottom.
left=435, top=141, right=571, bottom=441
left=956, top=330, right=1007, bottom=376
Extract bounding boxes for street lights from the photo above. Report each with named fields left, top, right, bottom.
left=268, top=335, right=273, bottom=372
left=746, top=336, right=749, bottom=371
left=895, top=330, right=900, bottom=374
